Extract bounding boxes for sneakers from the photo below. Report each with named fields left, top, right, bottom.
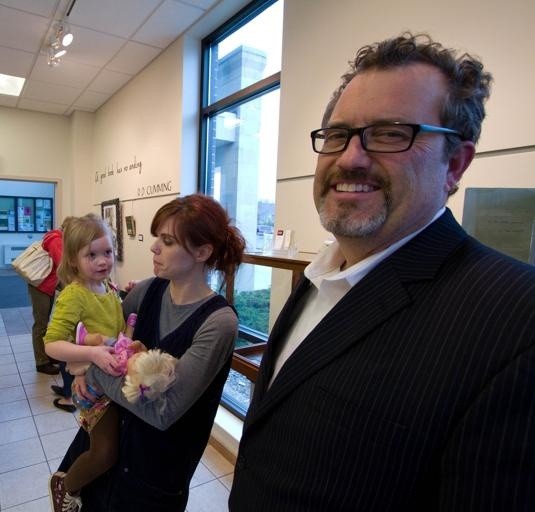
left=36, top=362, right=59, bottom=374
left=51, top=471, right=84, bottom=512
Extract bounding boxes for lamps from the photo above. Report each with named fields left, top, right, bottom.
left=38, top=23, right=73, bottom=67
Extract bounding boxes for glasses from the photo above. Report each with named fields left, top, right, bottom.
left=311, top=121, right=464, bottom=154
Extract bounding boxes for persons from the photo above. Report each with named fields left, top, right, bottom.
left=27, top=216, right=74, bottom=375
left=54, top=193, right=248, bottom=512
left=229, top=25, right=535, bottom=512
left=41, top=211, right=127, bottom=511
left=49, top=358, right=78, bottom=413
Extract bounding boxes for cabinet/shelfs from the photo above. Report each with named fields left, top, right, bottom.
left=221, top=250, right=314, bottom=379
left=0, top=195, right=53, bottom=234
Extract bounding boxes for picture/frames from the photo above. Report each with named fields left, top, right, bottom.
left=100, top=197, right=123, bottom=260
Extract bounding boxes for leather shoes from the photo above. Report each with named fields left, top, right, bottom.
left=51, top=385, right=65, bottom=396
left=54, top=398, right=76, bottom=412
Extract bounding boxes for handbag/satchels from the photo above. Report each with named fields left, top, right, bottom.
left=10, top=233, right=61, bottom=288
